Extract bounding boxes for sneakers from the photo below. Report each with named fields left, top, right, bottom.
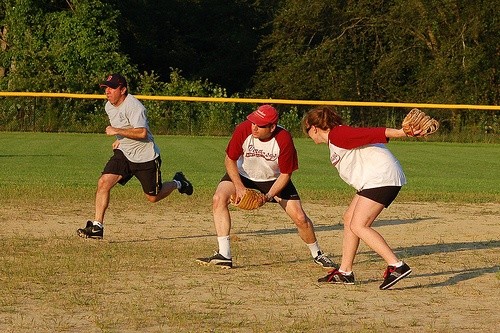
left=379, top=261, right=412, bottom=290
left=173, top=172, right=193, bottom=196
left=314, top=250, right=337, bottom=269
left=77, top=220, right=103, bottom=239
left=196, top=252, right=233, bottom=269
left=318, top=270, right=355, bottom=285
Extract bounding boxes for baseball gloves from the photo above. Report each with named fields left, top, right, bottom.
left=401, top=107, right=439, bottom=138
left=229, top=188, right=267, bottom=210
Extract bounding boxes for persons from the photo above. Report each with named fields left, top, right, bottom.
left=196, top=105, right=338, bottom=270
left=77, top=74, right=194, bottom=237
left=303, top=105, right=440, bottom=290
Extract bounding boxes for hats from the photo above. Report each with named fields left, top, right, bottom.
left=246, top=104, right=279, bottom=127
left=98, top=74, right=128, bottom=90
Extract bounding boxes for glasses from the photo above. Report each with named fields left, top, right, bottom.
left=305, top=126, right=311, bottom=133
